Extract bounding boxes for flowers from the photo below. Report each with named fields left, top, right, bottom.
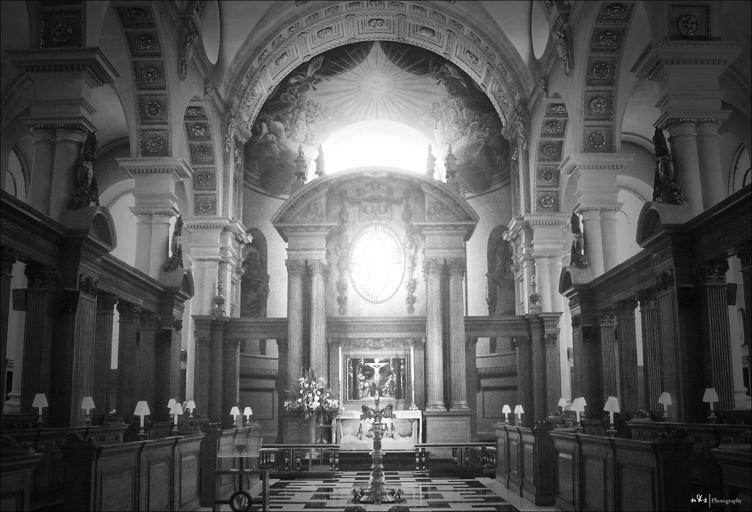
left=280, top=365, right=342, bottom=418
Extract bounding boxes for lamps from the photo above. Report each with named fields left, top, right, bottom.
left=501, top=404, right=525, bottom=426
left=133, top=401, right=151, bottom=433
left=701, top=388, right=721, bottom=416
left=80, top=397, right=95, bottom=420
left=558, top=396, right=588, bottom=425
left=230, top=405, right=254, bottom=427
left=167, top=399, right=196, bottom=429
left=658, top=393, right=673, bottom=418
left=32, top=392, right=48, bottom=421
left=602, top=397, right=619, bottom=428
